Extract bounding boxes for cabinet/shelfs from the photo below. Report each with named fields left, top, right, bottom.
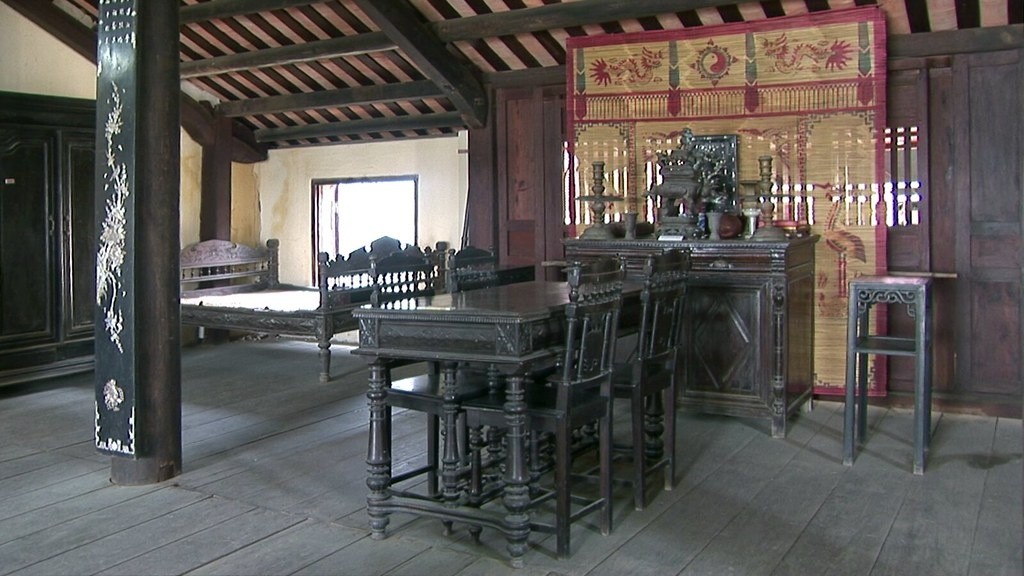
left=0, top=89, right=95, bottom=390
left=843, top=273, right=933, bottom=475
left=561, top=233, right=819, bottom=438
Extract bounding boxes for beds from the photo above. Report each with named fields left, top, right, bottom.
left=179, top=237, right=445, bottom=384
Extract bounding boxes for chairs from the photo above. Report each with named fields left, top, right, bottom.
left=448, top=247, right=558, bottom=461
left=552, top=246, right=691, bottom=512
left=462, top=254, right=628, bottom=559
left=366, top=244, right=505, bottom=535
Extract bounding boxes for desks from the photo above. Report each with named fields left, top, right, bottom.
left=349, top=281, right=644, bottom=566
